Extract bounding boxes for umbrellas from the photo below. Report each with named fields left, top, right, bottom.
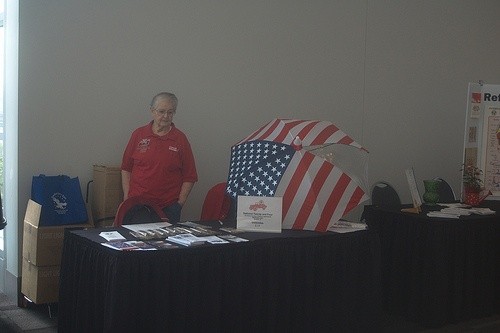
left=226, top=116, right=371, bottom=233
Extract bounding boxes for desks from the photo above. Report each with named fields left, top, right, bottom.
left=362, top=202, right=500, bottom=327
left=57, top=220, right=380, bottom=333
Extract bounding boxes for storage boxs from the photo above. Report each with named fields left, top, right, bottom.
left=23, top=199, right=96, bottom=266
left=90, top=166, right=123, bottom=221
left=22, top=258, right=61, bottom=304
left=96, top=218, right=115, bottom=227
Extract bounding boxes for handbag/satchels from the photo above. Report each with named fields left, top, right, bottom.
left=32, top=173, right=89, bottom=227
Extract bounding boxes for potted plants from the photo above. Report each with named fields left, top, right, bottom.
left=457, top=165, right=493, bottom=206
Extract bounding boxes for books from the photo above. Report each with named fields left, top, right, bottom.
left=405, top=168, right=423, bottom=209
left=120, top=222, right=172, bottom=232
left=325, top=220, right=368, bottom=233
left=427, top=202, right=497, bottom=219
left=99, top=230, right=126, bottom=241
left=199, top=236, right=229, bottom=245
left=220, top=234, right=249, bottom=243
left=101, top=241, right=157, bottom=252
left=166, top=233, right=206, bottom=247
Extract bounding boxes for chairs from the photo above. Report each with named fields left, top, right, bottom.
left=114, top=196, right=170, bottom=225
left=372, top=183, right=401, bottom=205
left=423, top=178, right=455, bottom=202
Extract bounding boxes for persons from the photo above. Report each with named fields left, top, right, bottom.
left=120, top=93, right=198, bottom=223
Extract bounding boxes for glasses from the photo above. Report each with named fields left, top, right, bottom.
left=153, top=108, right=175, bottom=115
